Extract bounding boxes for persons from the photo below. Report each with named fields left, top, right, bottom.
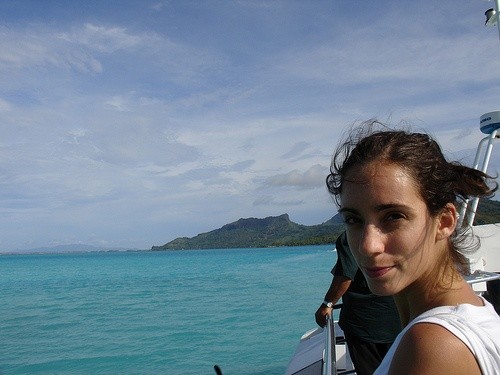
left=314, top=230, right=403, bottom=375
left=325, top=130, right=500, bottom=375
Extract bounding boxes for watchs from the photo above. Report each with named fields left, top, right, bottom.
left=323, top=298, right=336, bottom=309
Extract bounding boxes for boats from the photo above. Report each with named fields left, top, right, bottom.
left=283, top=109, right=500, bottom=375
